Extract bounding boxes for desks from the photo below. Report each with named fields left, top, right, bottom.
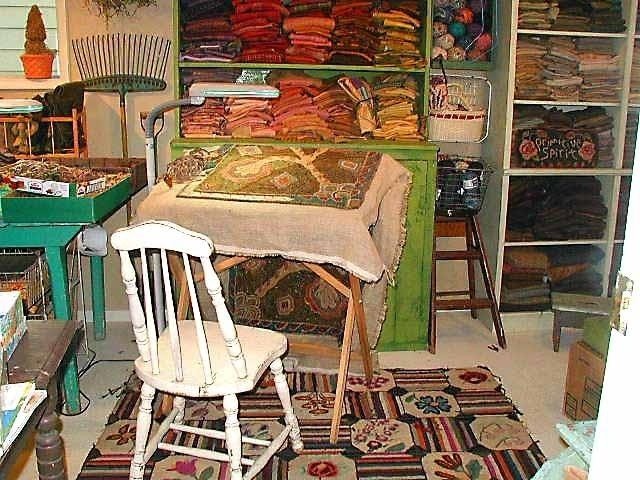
left=0, top=223, right=104, bottom=480
left=129, top=143, right=394, bottom=442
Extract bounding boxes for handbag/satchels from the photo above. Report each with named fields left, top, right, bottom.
left=516, top=127, right=599, bottom=168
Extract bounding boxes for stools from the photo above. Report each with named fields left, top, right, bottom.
left=550, top=289, right=611, bottom=353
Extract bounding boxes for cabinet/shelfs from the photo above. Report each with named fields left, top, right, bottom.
left=167, top=1, right=440, bottom=351
left=475, top=2, right=640, bottom=335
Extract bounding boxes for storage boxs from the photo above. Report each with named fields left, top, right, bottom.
left=560, top=339, right=606, bottom=422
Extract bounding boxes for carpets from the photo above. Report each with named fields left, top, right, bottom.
left=75, top=363, right=557, bottom=480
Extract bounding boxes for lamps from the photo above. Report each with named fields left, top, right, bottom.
left=146, top=83, right=278, bottom=193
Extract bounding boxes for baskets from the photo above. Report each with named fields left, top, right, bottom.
left=427, top=84, right=485, bottom=140
left=437, top=154, right=489, bottom=217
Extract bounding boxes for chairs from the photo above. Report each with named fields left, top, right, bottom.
left=109, top=221, right=305, bottom=480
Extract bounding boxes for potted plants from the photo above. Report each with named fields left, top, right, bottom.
left=19, top=4, right=59, bottom=79
